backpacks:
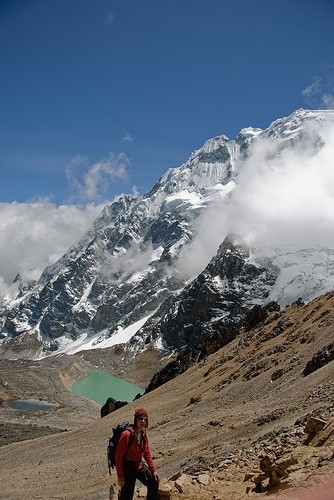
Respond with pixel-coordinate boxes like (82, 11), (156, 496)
(107, 420), (135, 475)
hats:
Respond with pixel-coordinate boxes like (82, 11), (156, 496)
(133, 408), (148, 429)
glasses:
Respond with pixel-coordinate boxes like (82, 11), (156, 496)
(138, 417), (147, 422)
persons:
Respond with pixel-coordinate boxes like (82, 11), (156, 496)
(115, 407), (160, 500)
